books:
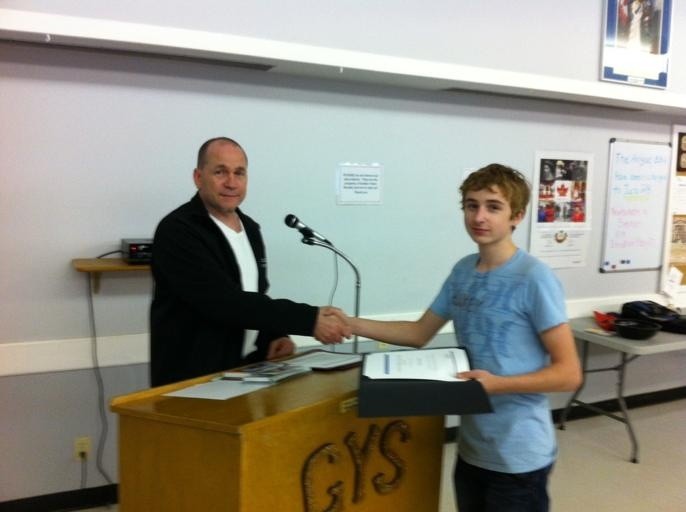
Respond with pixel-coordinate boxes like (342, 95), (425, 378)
(240, 362), (313, 383)
(220, 357), (286, 379)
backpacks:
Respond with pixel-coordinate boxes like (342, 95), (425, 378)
(625, 299), (686, 335)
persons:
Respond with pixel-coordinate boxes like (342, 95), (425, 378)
(309, 161), (586, 511)
(147, 136), (353, 398)
(538, 160), (585, 223)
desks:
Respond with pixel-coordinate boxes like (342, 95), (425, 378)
(556, 315), (686, 463)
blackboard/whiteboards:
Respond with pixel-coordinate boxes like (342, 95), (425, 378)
(599, 138), (672, 274)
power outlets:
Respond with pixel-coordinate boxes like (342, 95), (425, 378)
(76, 437), (90, 461)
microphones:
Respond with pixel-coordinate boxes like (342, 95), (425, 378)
(285, 214), (331, 245)
